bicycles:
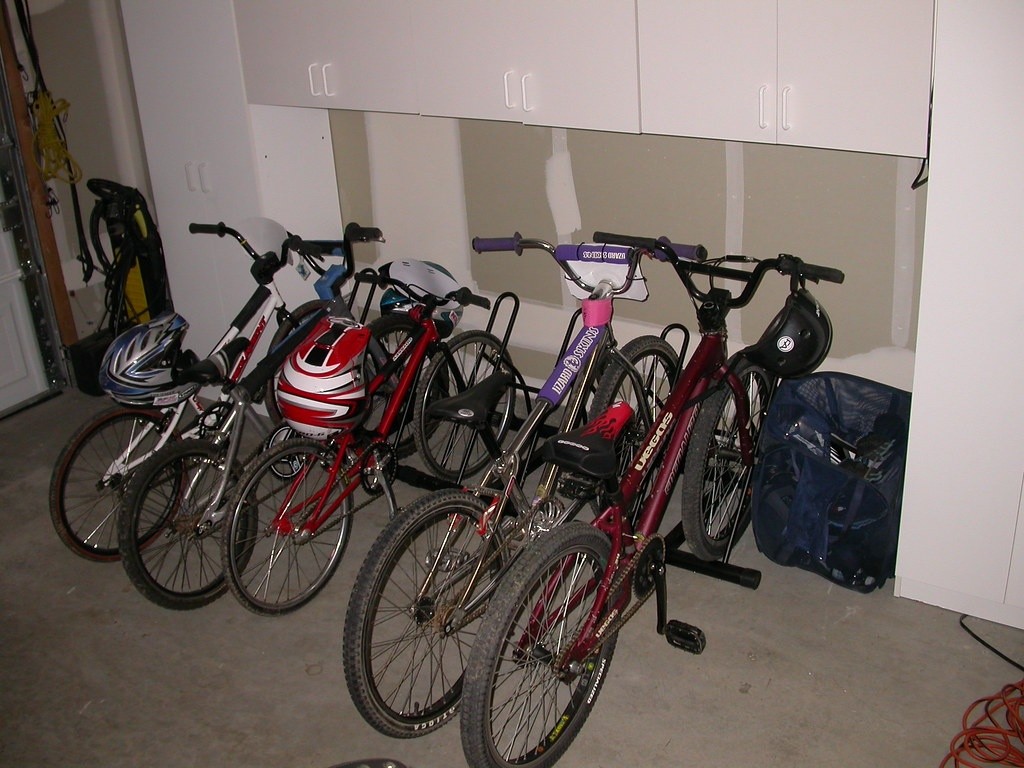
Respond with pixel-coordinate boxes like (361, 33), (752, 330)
(458, 230), (850, 767)
(340, 231), (708, 741)
(44, 220), (346, 562)
(220, 264), (522, 622)
(121, 221), (449, 611)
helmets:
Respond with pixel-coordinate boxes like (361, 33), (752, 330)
(272, 315), (370, 444)
(740, 288), (836, 380)
(98, 312), (205, 411)
(379, 261), (463, 337)
